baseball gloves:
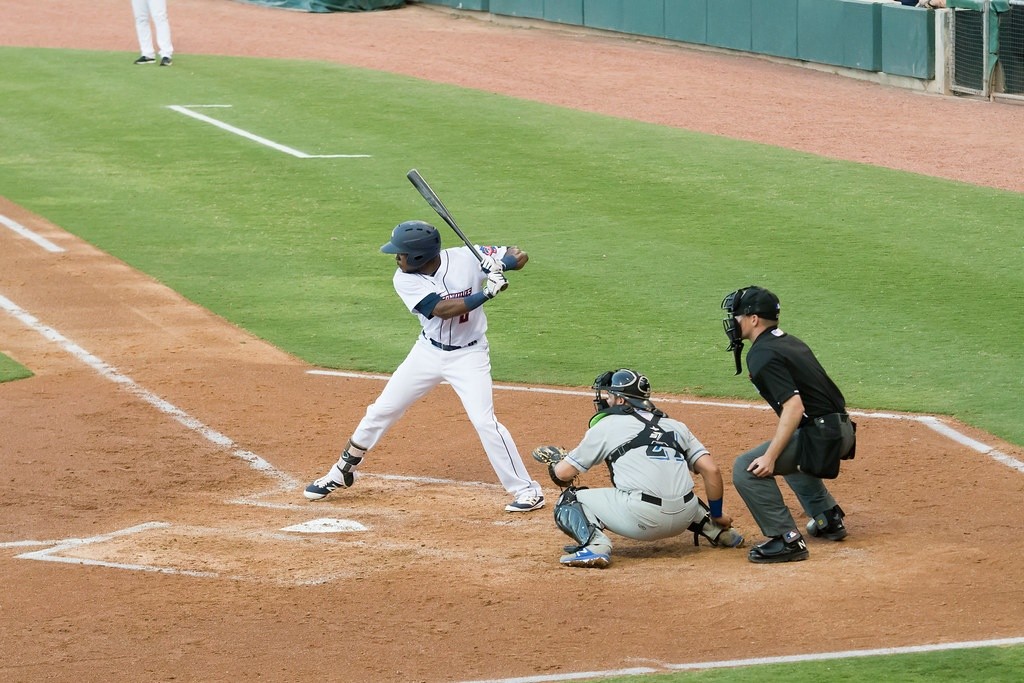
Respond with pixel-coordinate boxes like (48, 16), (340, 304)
(532, 445), (567, 465)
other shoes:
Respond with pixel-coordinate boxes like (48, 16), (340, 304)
(748, 534), (809, 563)
(806, 510), (847, 540)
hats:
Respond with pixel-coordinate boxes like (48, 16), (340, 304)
(730, 286), (780, 312)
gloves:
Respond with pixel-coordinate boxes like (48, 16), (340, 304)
(483, 271), (510, 299)
(479, 256), (506, 275)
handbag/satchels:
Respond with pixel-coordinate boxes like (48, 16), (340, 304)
(800, 416), (843, 479)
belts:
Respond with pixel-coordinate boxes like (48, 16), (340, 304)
(422, 330), (477, 352)
(839, 414), (849, 423)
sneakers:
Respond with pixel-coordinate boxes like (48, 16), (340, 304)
(135, 56), (156, 64)
(160, 57), (172, 66)
(716, 526), (744, 547)
(559, 545), (612, 568)
(504, 495), (545, 512)
(304, 473), (345, 500)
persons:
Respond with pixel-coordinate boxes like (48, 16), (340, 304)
(721, 285), (858, 564)
(303, 221), (546, 512)
(533, 367), (745, 569)
(131, 0), (174, 68)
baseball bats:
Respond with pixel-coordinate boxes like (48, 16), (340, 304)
(406, 169), (509, 291)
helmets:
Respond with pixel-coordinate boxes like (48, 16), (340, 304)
(594, 366), (651, 399)
(379, 220), (441, 269)
